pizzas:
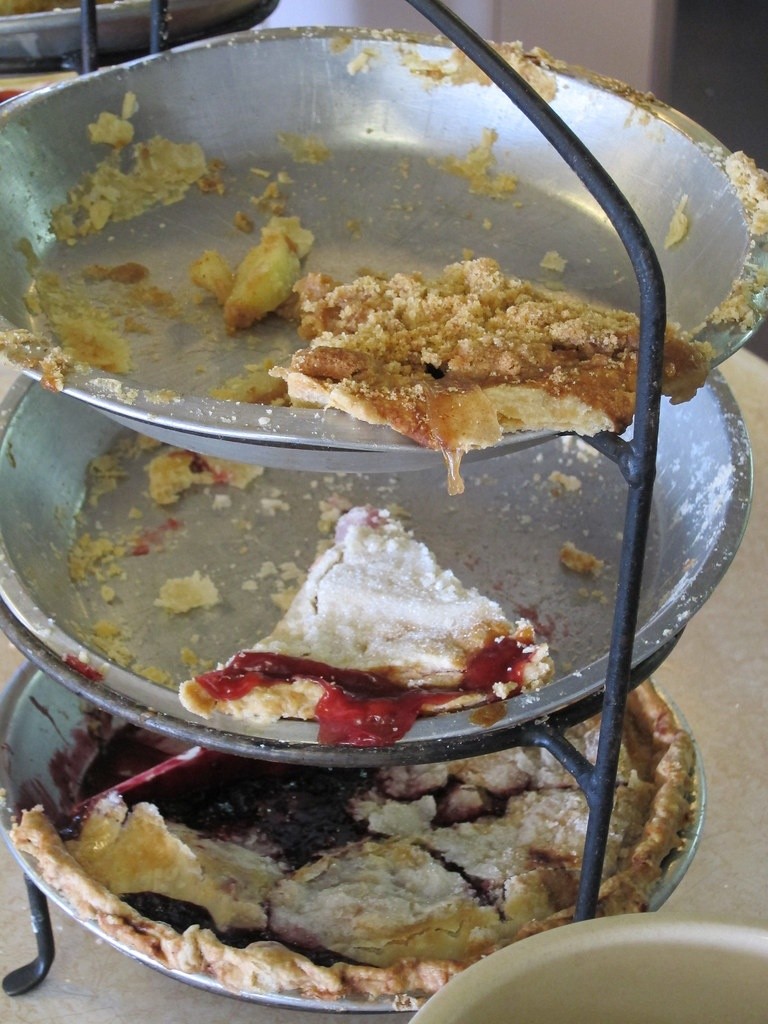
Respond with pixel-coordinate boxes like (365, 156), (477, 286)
(177, 504), (556, 746)
(267, 253), (706, 451)
(11, 673), (693, 1001)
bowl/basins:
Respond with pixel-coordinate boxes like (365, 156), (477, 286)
(0, 369), (756, 746)
(0, 21), (768, 477)
(0, 661), (709, 1015)
(406, 911), (767, 1023)
